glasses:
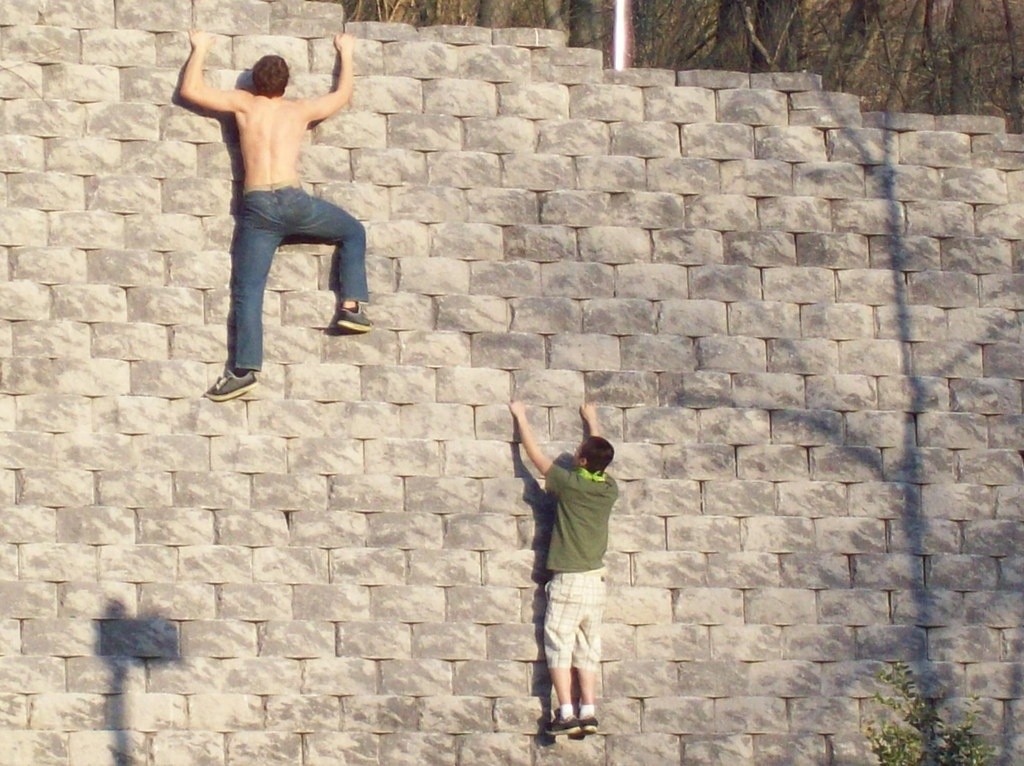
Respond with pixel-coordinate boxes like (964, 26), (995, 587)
(573, 443), (581, 455)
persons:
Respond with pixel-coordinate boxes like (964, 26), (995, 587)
(176, 30), (375, 402)
(506, 399), (620, 737)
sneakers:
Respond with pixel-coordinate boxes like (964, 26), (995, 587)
(544, 715), (582, 736)
(569, 716), (599, 737)
(337, 302), (374, 333)
(204, 370), (259, 401)
